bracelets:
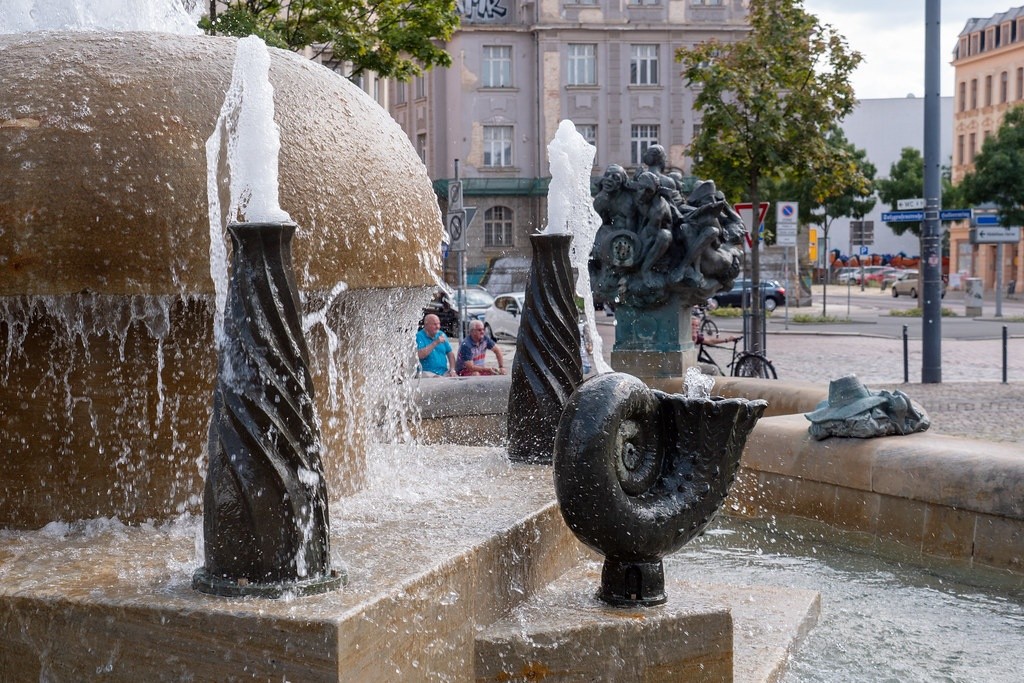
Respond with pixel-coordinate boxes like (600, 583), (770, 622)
(725, 338), (728, 343)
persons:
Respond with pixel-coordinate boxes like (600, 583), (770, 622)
(690, 317), (737, 376)
(417, 313), (455, 378)
(454, 320), (507, 377)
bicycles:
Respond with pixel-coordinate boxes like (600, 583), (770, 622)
(691, 304), (718, 341)
(697, 334), (779, 381)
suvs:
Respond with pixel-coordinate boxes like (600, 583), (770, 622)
(425, 282), (495, 339)
(699, 278), (787, 311)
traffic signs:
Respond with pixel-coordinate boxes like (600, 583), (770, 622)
(975, 225), (1020, 243)
(897, 198), (925, 211)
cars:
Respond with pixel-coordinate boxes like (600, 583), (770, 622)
(484, 291), (587, 343)
(829, 267), (903, 287)
(891, 272), (946, 298)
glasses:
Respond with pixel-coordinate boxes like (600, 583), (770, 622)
(474, 328), (485, 331)
